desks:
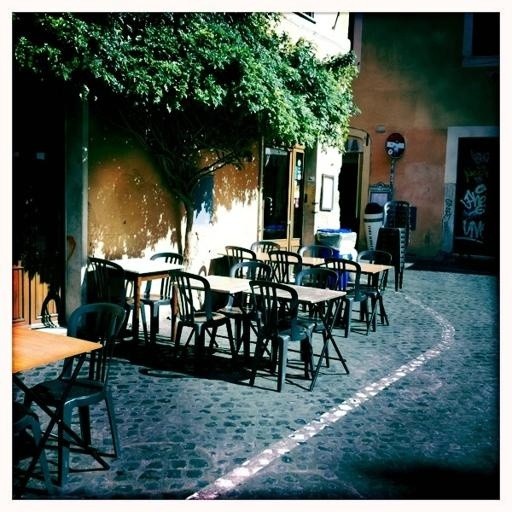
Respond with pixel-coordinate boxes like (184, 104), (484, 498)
(12, 323), (110, 500)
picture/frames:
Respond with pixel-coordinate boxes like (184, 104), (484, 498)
(320, 174), (335, 212)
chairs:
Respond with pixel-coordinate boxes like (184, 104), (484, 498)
(372, 200), (411, 292)
(12, 400), (57, 500)
(23, 302), (128, 490)
